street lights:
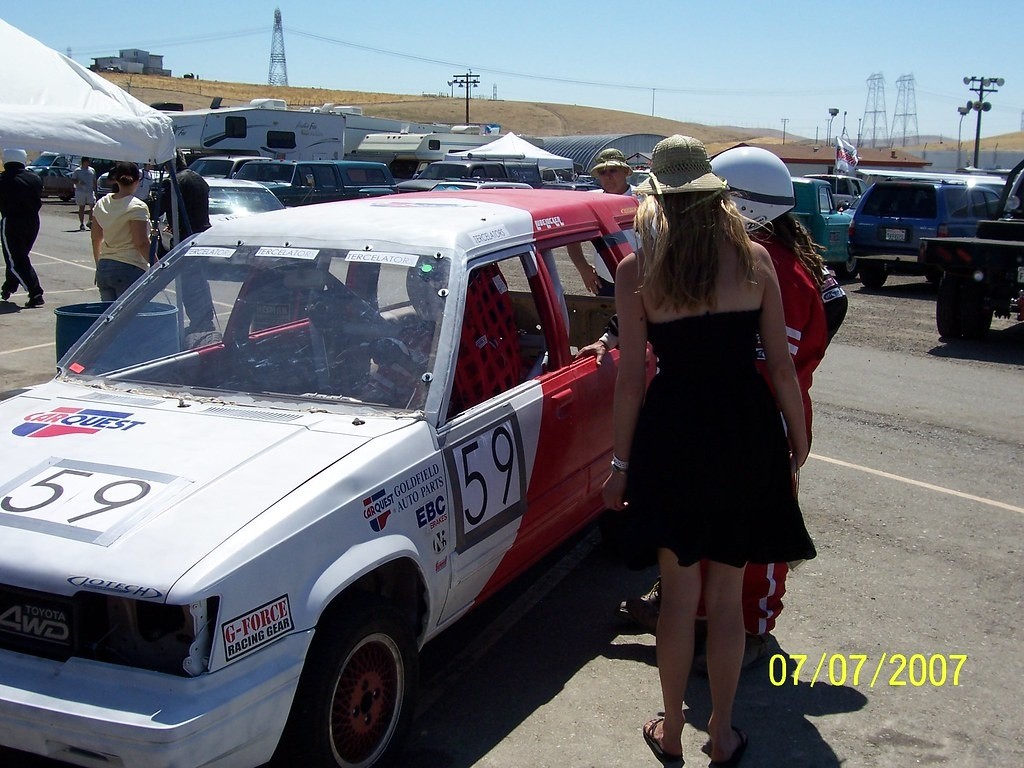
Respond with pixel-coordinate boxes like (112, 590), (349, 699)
(446, 75), (482, 124)
(827, 108), (840, 147)
(957, 107), (972, 171)
(963, 75), (1006, 166)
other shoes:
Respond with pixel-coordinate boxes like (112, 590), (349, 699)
(25, 294), (45, 307)
(86, 221), (92, 229)
(695, 634), (770, 672)
(1, 289), (11, 300)
(614, 575), (660, 629)
(80, 224), (85, 230)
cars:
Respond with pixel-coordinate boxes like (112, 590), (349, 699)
(0, 188), (656, 768)
(803, 173), (872, 220)
(788, 176), (858, 281)
(30, 107), (651, 275)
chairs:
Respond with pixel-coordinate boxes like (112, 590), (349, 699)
(405, 256), (525, 417)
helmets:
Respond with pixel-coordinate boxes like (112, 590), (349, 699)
(708, 146), (796, 231)
(2, 149), (28, 167)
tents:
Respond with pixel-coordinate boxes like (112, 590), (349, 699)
(0, 20), (175, 163)
(444, 132), (573, 172)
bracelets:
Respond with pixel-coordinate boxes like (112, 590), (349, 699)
(612, 453), (629, 473)
(75, 181), (80, 185)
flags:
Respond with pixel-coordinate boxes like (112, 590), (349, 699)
(836, 137), (859, 177)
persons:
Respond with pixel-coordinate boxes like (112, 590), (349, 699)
(575, 220), (848, 368)
(598, 133), (817, 768)
(696, 146), (824, 680)
(567, 147), (642, 297)
(158, 146), (216, 334)
(133, 164), (153, 200)
(0, 149), (45, 308)
(105, 161), (122, 193)
(91, 162), (159, 301)
(71, 156), (97, 232)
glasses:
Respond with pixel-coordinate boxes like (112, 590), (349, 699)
(598, 168), (623, 175)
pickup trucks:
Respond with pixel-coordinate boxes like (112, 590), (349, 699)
(918, 159), (1024, 340)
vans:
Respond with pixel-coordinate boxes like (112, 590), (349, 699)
(847, 177), (1003, 288)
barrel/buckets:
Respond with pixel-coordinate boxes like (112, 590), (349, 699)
(54, 300), (181, 375)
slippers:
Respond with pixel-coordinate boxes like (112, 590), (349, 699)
(709, 726), (749, 768)
(643, 717), (685, 762)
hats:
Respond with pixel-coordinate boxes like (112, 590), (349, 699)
(632, 134), (730, 194)
(591, 148), (633, 179)
